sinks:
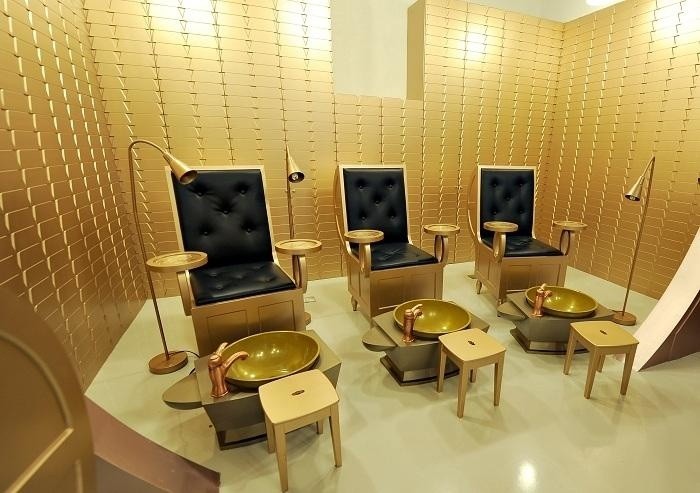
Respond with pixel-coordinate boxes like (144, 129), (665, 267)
(525, 286), (598, 318)
(224, 330), (320, 392)
(394, 298), (471, 339)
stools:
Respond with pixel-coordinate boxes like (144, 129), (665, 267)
(258, 368), (343, 492)
(436, 326), (506, 417)
(564, 319), (639, 398)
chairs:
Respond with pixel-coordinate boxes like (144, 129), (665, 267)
(465, 165), (588, 317)
(333, 163), (460, 327)
(163, 164), (307, 355)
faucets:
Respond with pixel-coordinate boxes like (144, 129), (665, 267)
(206, 343), (249, 397)
(534, 283), (552, 316)
(402, 303), (422, 342)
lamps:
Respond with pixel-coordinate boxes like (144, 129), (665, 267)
(128, 139), (198, 376)
(285, 145), (311, 323)
(612, 155), (656, 325)
